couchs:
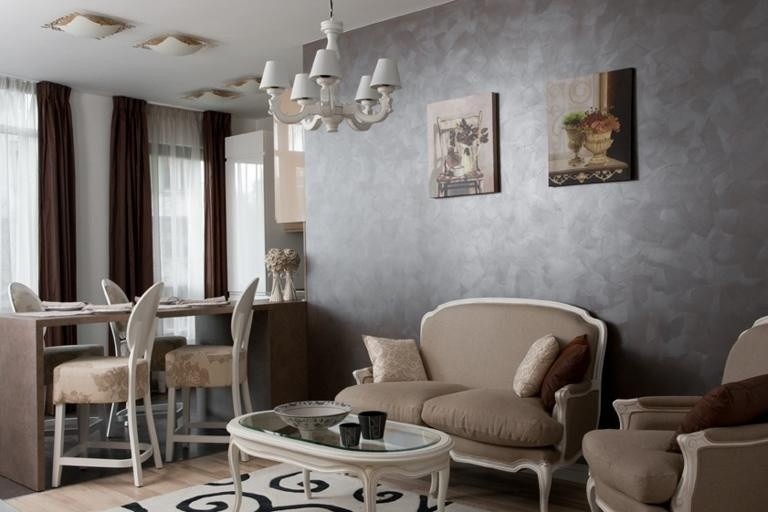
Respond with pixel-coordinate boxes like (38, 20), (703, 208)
(582, 315), (768, 511)
(335, 297), (608, 511)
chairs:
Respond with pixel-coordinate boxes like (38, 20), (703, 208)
(101, 278), (187, 437)
(53, 282), (163, 487)
(9, 281), (105, 442)
(166, 278), (259, 462)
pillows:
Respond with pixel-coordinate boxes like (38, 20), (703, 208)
(361, 335), (428, 383)
(542, 334), (591, 409)
(665, 375), (768, 453)
(513, 332), (560, 398)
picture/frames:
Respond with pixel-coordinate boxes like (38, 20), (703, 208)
(425, 92), (497, 199)
(548, 67), (636, 187)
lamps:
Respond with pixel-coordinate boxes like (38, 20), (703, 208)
(221, 78), (267, 94)
(132, 32), (218, 57)
(259, 1), (402, 133)
(39, 11), (136, 40)
(179, 90), (245, 105)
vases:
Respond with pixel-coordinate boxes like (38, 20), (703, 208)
(284, 271), (297, 301)
(270, 270), (283, 302)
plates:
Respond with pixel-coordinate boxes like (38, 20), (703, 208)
(158, 298), (178, 305)
(187, 302), (231, 309)
(44, 306), (84, 312)
(90, 310), (131, 315)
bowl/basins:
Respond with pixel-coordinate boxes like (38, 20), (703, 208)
(273, 399), (352, 433)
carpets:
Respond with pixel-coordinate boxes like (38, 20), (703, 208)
(107, 463), (495, 512)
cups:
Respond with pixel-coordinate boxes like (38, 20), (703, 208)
(338, 422), (362, 449)
(357, 410), (387, 441)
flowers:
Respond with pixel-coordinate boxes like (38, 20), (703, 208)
(283, 248), (301, 272)
(264, 248), (287, 273)
